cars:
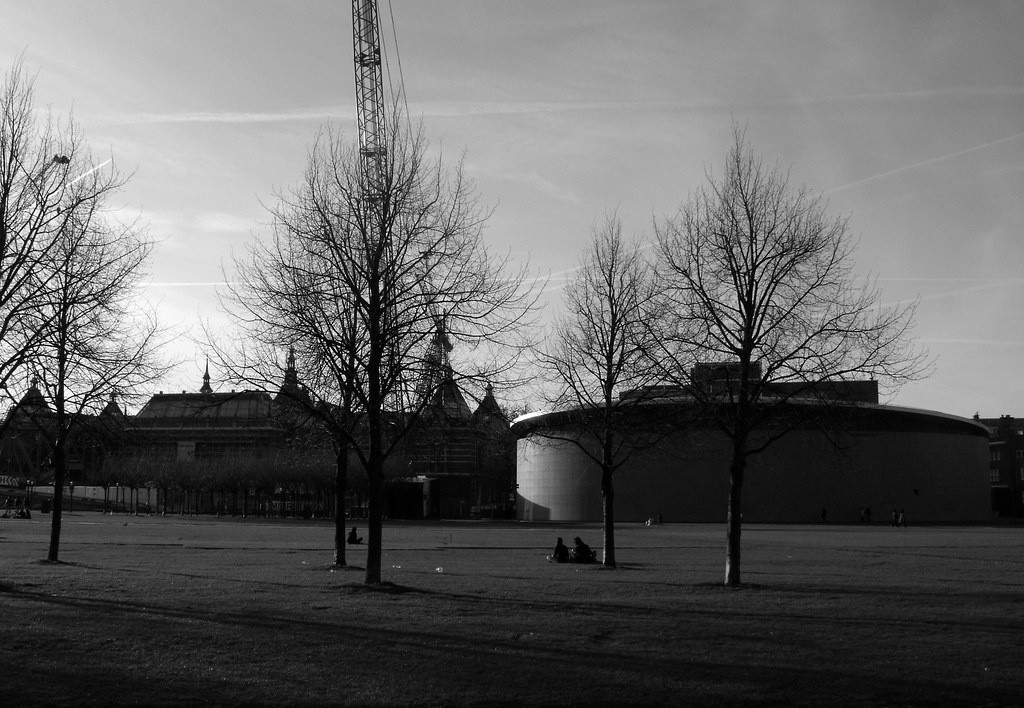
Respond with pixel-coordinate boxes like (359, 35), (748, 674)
(345, 506), (368, 517)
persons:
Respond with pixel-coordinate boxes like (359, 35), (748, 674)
(740, 512), (744, 523)
(859, 505), (871, 525)
(551, 537), (592, 562)
(658, 512), (663, 525)
(347, 527), (363, 544)
(888, 508), (907, 527)
(5, 496), (31, 519)
(821, 505), (828, 524)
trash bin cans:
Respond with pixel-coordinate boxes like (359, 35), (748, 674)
(41, 499), (50, 514)
